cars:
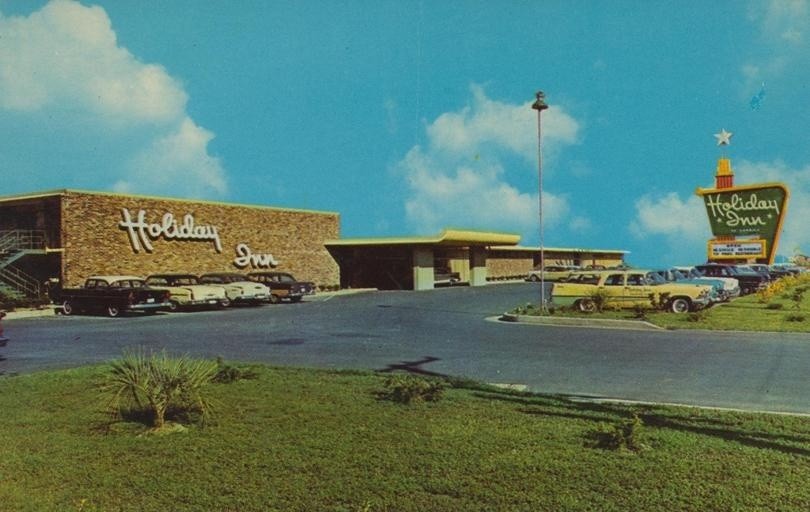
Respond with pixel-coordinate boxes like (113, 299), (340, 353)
(246, 272), (316, 304)
(200, 272), (271, 308)
(144, 274), (229, 312)
(549, 269), (711, 315)
(48, 276), (173, 318)
(528, 262), (810, 308)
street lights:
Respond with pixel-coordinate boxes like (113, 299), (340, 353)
(532, 92), (548, 314)
